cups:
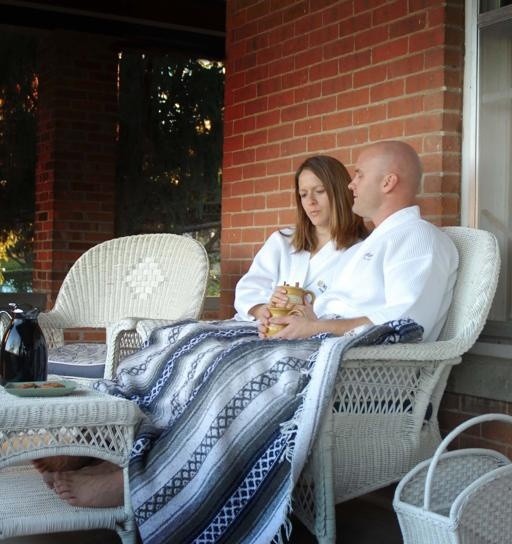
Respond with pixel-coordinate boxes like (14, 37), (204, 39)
(266, 306), (305, 338)
(275, 286), (316, 310)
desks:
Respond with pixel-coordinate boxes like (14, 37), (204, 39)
(0, 385), (141, 544)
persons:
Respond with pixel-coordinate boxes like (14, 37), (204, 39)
(41, 139), (462, 511)
(28, 152), (374, 468)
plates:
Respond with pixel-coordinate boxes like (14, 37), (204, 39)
(3, 378), (78, 398)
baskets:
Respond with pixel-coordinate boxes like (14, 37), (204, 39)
(392, 414), (511, 544)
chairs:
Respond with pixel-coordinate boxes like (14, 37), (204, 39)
(136, 225), (502, 544)
(0, 232), (211, 380)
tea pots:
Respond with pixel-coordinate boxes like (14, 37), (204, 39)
(6, 304), (44, 382)
